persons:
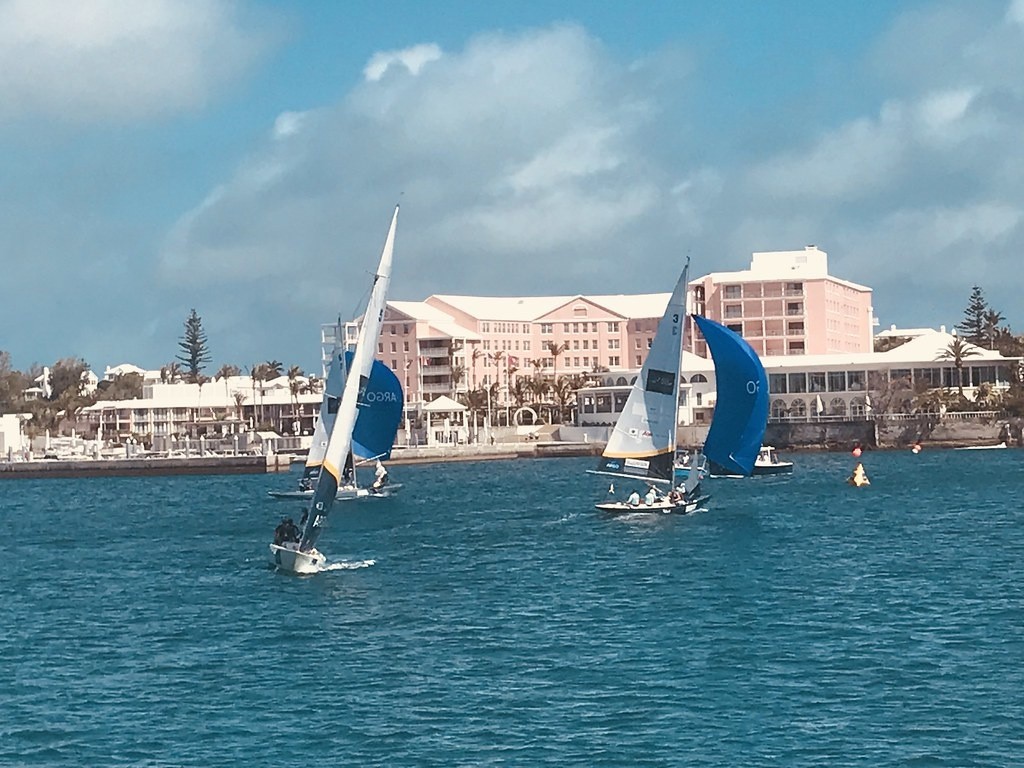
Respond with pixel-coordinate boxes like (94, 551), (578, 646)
(645, 486), (657, 506)
(275, 518), (300, 546)
(667, 482), (686, 503)
(626, 490), (640, 506)
(299, 477), (313, 492)
(759, 451), (779, 464)
(676, 453), (690, 468)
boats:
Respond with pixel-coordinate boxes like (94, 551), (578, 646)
(673, 448), (709, 478)
(708, 442), (795, 479)
(846, 462), (872, 489)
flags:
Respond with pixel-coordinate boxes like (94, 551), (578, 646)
(423, 357), (432, 367)
(508, 354), (518, 366)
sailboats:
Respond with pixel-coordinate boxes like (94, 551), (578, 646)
(583, 257), (770, 516)
(267, 202), (402, 576)
(266, 312), (402, 502)
